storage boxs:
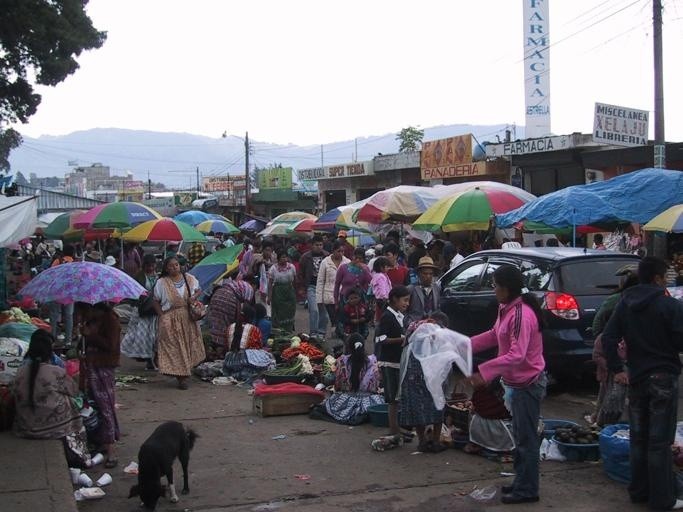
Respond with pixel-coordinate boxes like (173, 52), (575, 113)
(251, 392), (325, 420)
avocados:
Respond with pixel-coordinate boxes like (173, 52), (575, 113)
(551, 422), (600, 443)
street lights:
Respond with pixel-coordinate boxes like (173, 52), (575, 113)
(221, 130), (249, 222)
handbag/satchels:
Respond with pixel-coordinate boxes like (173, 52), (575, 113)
(138, 296), (156, 315)
(189, 300), (207, 320)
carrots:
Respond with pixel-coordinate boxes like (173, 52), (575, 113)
(282, 341), (322, 358)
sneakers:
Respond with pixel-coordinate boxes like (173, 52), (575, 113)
(502, 496), (539, 503)
(501, 485), (515, 493)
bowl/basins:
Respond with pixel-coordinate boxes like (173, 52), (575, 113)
(264, 374), (296, 384)
(367, 405), (401, 426)
(542, 419), (578, 434)
(550, 434), (600, 461)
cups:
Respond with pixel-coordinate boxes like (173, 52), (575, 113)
(95, 473), (112, 486)
(89, 452), (103, 465)
(78, 473), (92, 487)
(68, 468), (79, 484)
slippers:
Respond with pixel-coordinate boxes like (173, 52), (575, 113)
(106, 456), (118, 468)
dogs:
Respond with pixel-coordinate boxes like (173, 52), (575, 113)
(127, 420), (202, 512)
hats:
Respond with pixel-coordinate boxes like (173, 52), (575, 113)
(417, 257), (438, 269)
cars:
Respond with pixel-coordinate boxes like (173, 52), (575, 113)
(137, 236), (223, 271)
(429, 241), (643, 395)
